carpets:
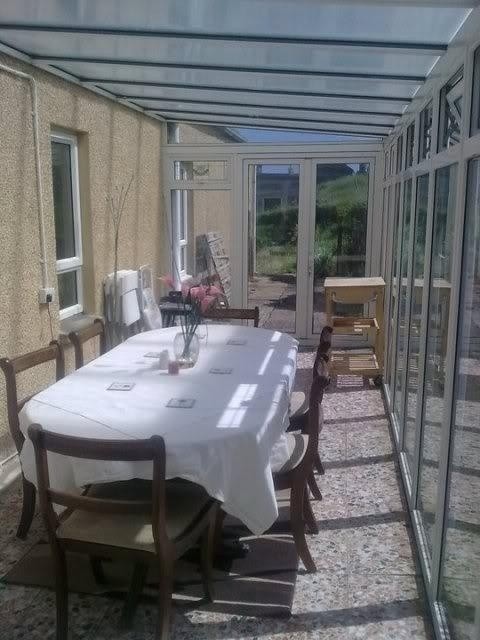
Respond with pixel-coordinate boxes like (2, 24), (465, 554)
(1, 522), (302, 620)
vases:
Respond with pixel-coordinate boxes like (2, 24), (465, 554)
(173, 333), (200, 369)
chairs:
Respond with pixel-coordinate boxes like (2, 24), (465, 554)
(0, 339), (87, 540)
(208, 306), (261, 329)
(289, 325), (334, 500)
(218, 353), (329, 576)
(102, 264), (162, 349)
(68, 316), (110, 370)
(27, 421), (221, 640)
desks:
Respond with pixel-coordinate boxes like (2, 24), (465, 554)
(159, 301), (194, 327)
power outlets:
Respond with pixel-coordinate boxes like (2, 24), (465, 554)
(39, 288), (55, 304)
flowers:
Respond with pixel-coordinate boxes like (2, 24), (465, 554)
(160, 273), (227, 358)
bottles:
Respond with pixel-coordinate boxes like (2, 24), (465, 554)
(159, 349), (181, 376)
